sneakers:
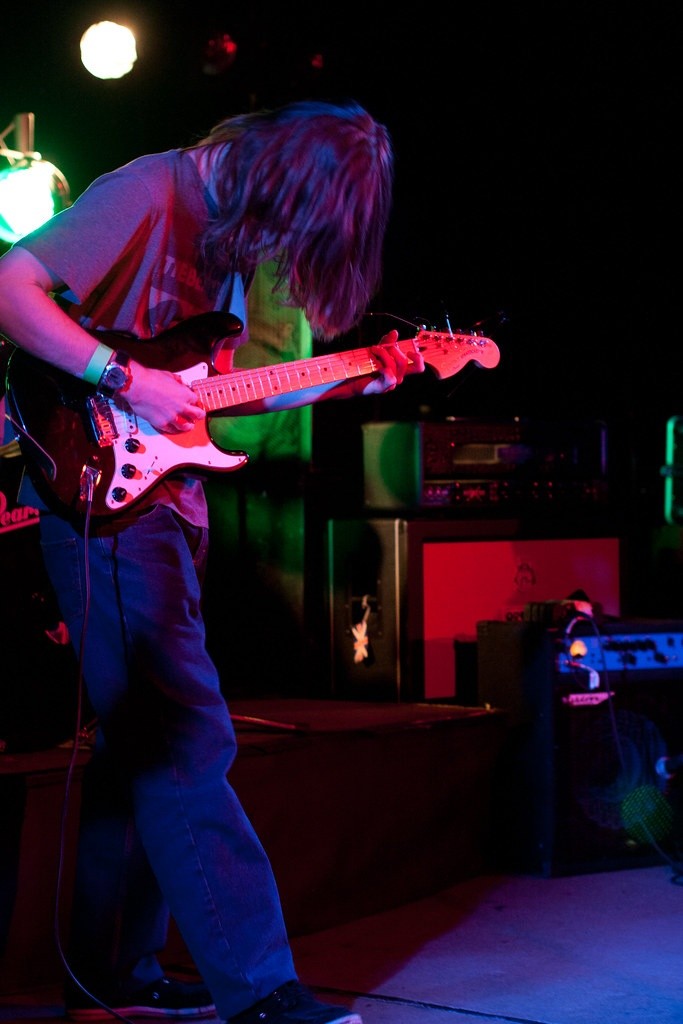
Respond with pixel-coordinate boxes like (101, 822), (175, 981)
(218, 979), (362, 1024)
(62, 970), (215, 1021)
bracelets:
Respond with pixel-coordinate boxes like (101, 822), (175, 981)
(82, 342), (114, 386)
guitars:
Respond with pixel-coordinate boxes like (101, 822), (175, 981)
(5, 309), (502, 524)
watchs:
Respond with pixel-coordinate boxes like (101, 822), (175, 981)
(99, 349), (131, 399)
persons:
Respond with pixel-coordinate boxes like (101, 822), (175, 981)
(0, 95), (425, 1024)
